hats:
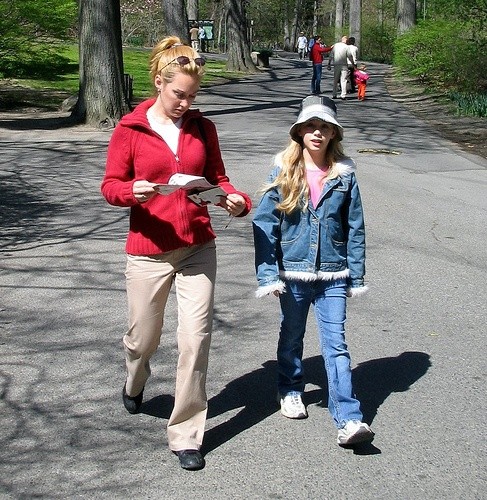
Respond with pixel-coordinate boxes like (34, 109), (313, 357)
(289, 95), (344, 148)
(300, 31), (305, 34)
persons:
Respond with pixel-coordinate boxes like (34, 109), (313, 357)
(353, 64), (369, 100)
(101, 35), (252, 470)
(197, 25), (206, 53)
(295, 31), (308, 61)
(327, 35), (356, 100)
(189, 23), (199, 53)
(252, 95), (376, 446)
(309, 35), (334, 95)
(309, 35), (314, 52)
(347, 37), (358, 94)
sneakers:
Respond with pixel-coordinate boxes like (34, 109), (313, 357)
(172, 449), (203, 469)
(337, 419), (375, 447)
(276, 390), (308, 419)
(122, 380), (144, 414)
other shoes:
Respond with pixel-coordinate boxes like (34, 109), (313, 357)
(332, 95), (337, 99)
(340, 97), (345, 100)
(312, 90), (323, 94)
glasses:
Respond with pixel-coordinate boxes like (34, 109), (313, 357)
(157, 56), (207, 73)
